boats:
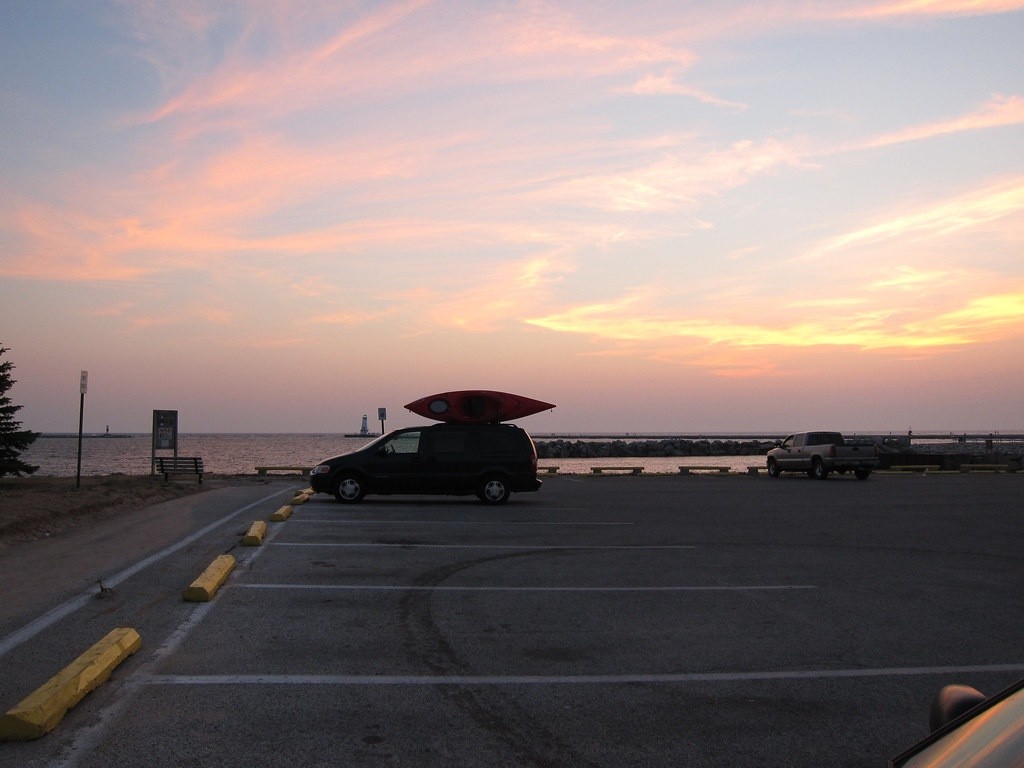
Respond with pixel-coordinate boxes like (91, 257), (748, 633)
(343, 415), (382, 438)
(403, 389), (557, 424)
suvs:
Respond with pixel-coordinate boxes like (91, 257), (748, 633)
(309, 422), (543, 505)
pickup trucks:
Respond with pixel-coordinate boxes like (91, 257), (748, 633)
(766, 431), (880, 480)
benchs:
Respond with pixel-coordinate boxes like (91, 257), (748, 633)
(155, 457), (213, 483)
(537, 464), (768, 476)
(254, 466), (314, 477)
(891, 462), (1024, 473)
